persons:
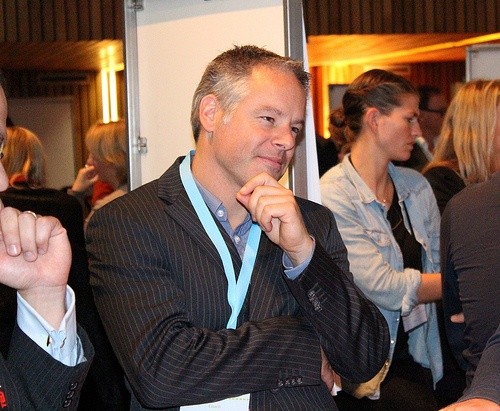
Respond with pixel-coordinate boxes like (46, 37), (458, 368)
(85, 45), (390, 411)
(319, 69), (444, 411)
(441, 174), (500, 396)
(66, 119), (130, 231)
(423, 80), (500, 214)
(0, 84), (95, 411)
(1, 126), (66, 198)
(326, 108), (350, 165)
(392, 84), (445, 173)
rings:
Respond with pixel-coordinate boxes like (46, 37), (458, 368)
(23, 209), (39, 219)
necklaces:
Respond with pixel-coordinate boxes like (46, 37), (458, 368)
(378, 195), (390, 204)
